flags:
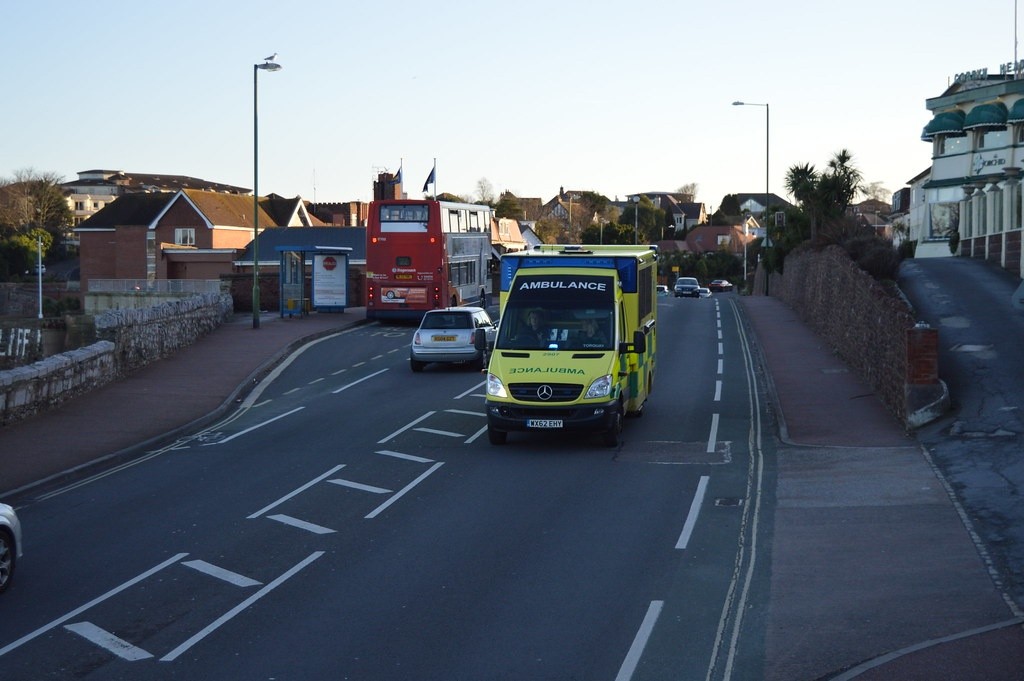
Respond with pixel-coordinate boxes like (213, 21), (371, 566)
(389, 166), (402, 184)
(422, 166), (436, 192)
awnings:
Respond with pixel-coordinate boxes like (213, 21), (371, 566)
(1006, 97), (1024, 123)
(963, 101), (1008, 129)
(920, 108), (966, 140)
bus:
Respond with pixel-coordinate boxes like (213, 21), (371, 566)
(364, 198), (493, 323)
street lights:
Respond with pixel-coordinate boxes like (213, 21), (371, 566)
(252, 63), (284, 328)
(567, 192), (581, 224)
(732, 100), (770, 295)
(599, 217), (610, 245)
(634, 196), (640, 245)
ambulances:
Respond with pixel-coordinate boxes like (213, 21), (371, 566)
(484, 243), (658, 448)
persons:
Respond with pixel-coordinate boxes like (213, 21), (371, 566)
(577, 315), (608, 344)
(510, 309), (549, 348)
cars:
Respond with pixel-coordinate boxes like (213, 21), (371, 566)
(409, 307), (493, 372)
(708, 279), (733, 292)
(699, 288), (712, 298)
(35, 264), (46, 276)
(657, 285), (670, 296)
(674, 276), (700, 298)
(0, 503), (23, 589)
